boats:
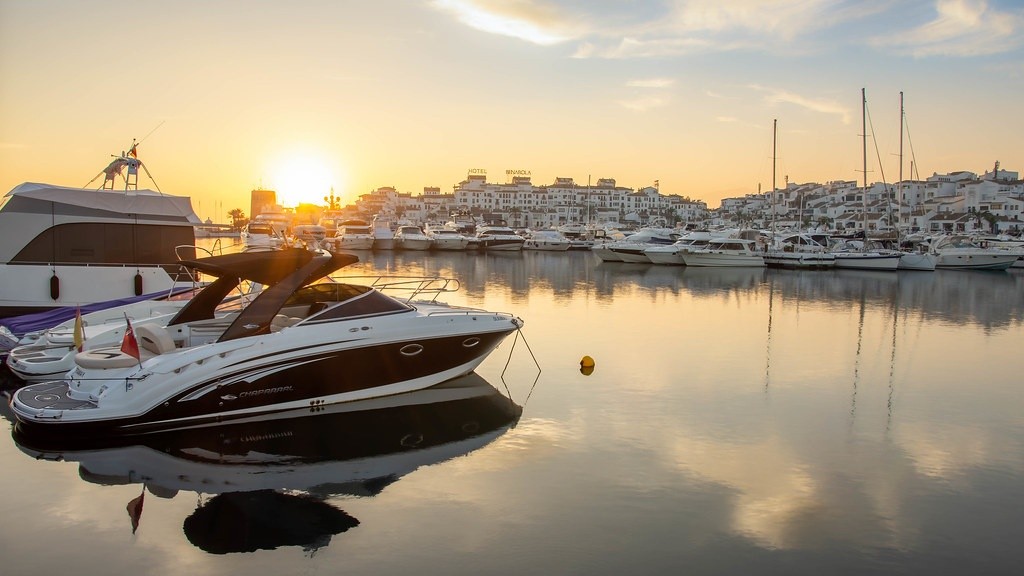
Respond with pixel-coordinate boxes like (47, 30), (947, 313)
(609, 229), (683, 262)
(644, 231), (732, 265)
(515, 225), (623, 248)
(592, 240), (624, 261)
(467, 218), (524, 250)
(9, 247), (524, 438)
(979, 236), (1024, 247)
(237, 207), (341, 249)
(12, 371), (538, 554)
(524, 230), (570, 250)
(0, 139), (199, 317)
(680, 222), (935, 235)
(929, 234), (1024, 269)
(445, 210), (477, 249)
(394, 226), (433, 250)
(0, 280), (269, 383)
(423, 223), (468, 250)
(681, 238), (768, 267)
(334, 220), (374, 250)
(370, 215), (396, 250)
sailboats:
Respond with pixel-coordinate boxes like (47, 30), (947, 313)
(763, 118), (835, 268)
(865, 91), (939, 272)
(830, 88), (903, 270)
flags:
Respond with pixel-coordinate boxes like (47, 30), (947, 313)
(130, 144), (137, 158)
(73, 309), (82, 352)
(120, 322), (140, 360)
